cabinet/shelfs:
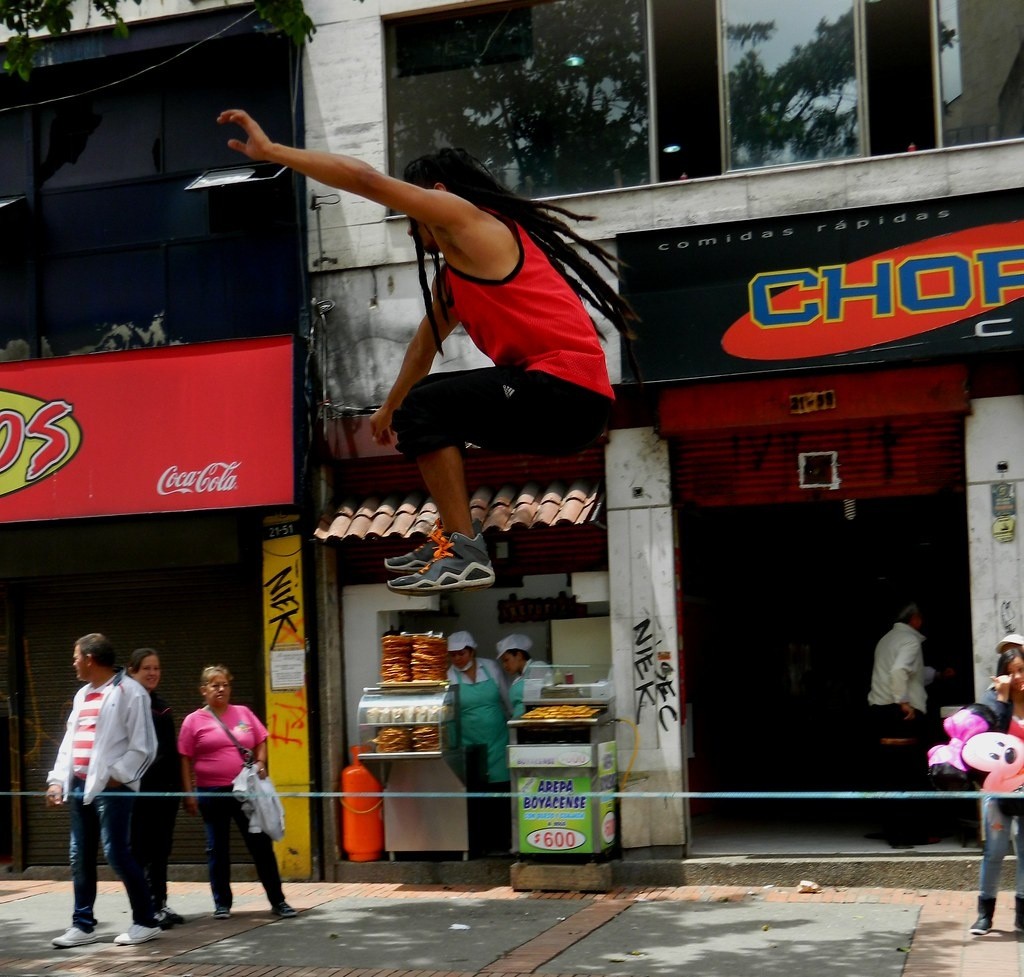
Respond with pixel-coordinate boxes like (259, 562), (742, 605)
(355, 685), (459, 760)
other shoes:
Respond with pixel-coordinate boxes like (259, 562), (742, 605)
(213, 906), (230, 918)
(271, 901), (297, 916)
(145, 905), (185, 930)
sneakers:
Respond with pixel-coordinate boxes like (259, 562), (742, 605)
(384, 518), (483, 574)
(113, 924), (162, 944)
(51, 926), (99, 947)
(387, 532), (497, 596)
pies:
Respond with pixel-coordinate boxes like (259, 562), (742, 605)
(521, 704), (600, 718)
(371, 725), (449, 753)
(379, 634), (449, 682)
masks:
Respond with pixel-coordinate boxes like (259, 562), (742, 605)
(454, 660), (473, 671)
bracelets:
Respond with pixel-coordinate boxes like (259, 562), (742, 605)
(254, 760), (266, 767)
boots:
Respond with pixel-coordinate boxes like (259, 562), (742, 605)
(1013, 896), (1024, 933)
(969, 895), (996, 934)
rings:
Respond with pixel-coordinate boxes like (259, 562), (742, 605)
(260, 768), (265, 772)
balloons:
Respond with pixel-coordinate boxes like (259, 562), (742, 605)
(925, 703), (1024, 819)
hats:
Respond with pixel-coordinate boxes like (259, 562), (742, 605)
(496, 633), (534, 659)
(995, 634), (1024, 654)
(447, 631), (477, 651)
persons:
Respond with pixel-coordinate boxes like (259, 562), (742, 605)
(125, 648), (185, 931)
(45, 633), (163, 947)
(446, 630), (514, 855)
(177, 665), (298, 918)
(867, 603), (956, 845)
(494, 634), (555, 720)
(215, 109), (643, 597)
(968, 634), (1024, 935)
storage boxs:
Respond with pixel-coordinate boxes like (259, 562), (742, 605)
(541, 686), (592, 699)
(523, 678), (545, 699)
(592, 681), (611, 698)
